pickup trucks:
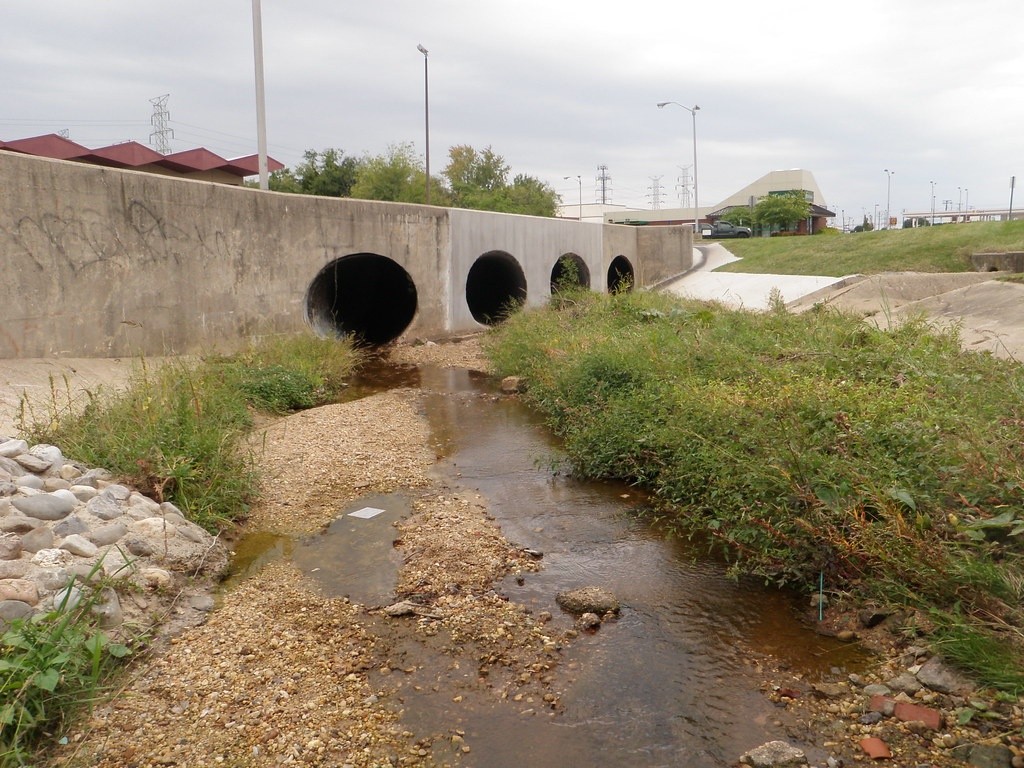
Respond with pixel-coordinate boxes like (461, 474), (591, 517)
(699, 220), (752, 238)
(693, 222), (713, 233)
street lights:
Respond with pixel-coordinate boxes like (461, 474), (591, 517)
(415, 44), (432, 205)
(884, 168), (895, 212)
(957, 186), (961, 224)
(929, 180), (937, 226)
(656, 100), (701, 233)
(964, 188), (968, 221)
(563, 175), (582, 221)
